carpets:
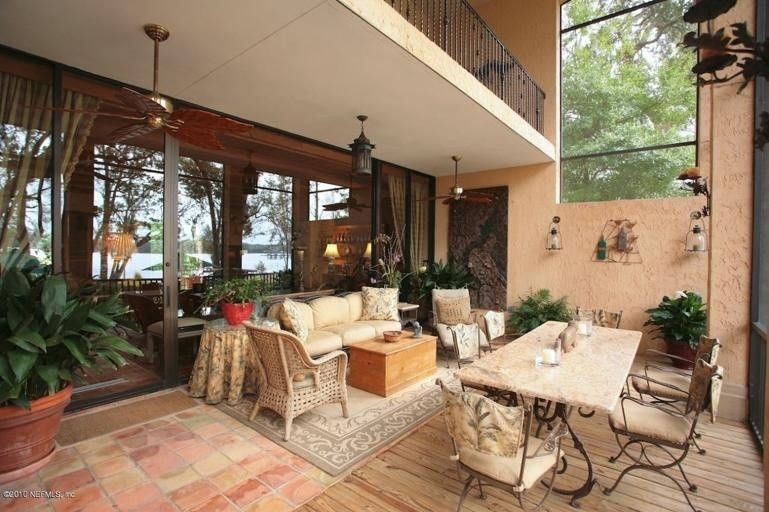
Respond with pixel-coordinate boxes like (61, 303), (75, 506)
(213, 364), (476, 477)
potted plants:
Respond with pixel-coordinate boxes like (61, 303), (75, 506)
(202, 278), (265, 325)
(0, 248), (145, 484)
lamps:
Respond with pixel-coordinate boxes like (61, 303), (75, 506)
(684, 216), (711, 252)
(241, 151), (258, 194)
(323, 243), (340, 273)
(545, 216), (562, 250)
(346, 115), (376, 177)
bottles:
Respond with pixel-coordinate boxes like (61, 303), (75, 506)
(618, 226), (628, 251)
(598, 236), (606, 259)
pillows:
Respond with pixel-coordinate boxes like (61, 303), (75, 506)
(266, 301), (313, 331)
(360, 287), (400, 322)
(280, 297), (308, 344)
(343, 291), (362, 322)
(309, 295), (349, 330)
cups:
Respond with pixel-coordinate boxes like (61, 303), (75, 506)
(534, 334), (562, 367)
(571, 309), (593, 337)
(415, 326), (422, 336)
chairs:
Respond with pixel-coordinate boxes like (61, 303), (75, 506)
(246, 326), (349, 442)
(123, 293), (164, 358)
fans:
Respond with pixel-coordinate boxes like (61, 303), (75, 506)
(416, 155), (495, 204)
(322, 176), (372, 212)
(22, 22), (256, 150)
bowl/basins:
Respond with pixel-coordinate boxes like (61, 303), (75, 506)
(383, 330), (401, 342)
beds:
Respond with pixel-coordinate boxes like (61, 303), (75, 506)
(146, 317), (207, 364)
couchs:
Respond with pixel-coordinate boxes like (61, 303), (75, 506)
(263, 291), (402, 369)
